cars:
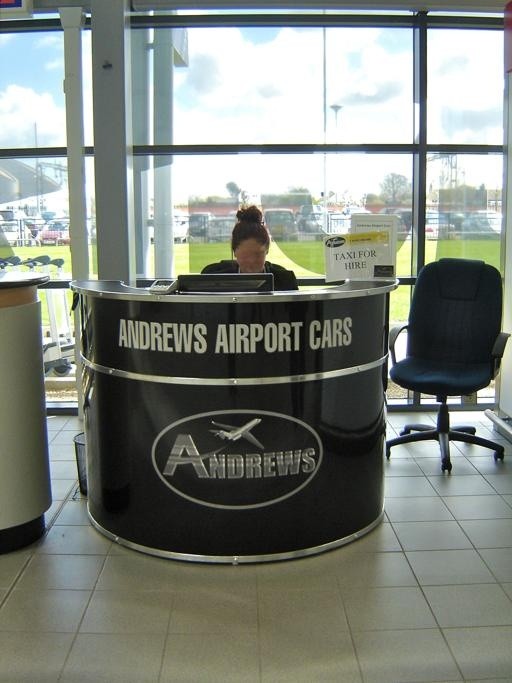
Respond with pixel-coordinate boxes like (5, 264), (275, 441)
(0, 210), (91, 245)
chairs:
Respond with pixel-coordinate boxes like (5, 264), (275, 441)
(386, 258), (511, 477)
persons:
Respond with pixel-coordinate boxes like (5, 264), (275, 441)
(200, 203), (298, 292)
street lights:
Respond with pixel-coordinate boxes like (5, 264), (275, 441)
(330, 101), (344, 202)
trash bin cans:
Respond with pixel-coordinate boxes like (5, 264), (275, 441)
(74, 432), (87, 495)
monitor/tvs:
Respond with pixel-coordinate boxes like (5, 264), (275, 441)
(177, 273), (275, 293)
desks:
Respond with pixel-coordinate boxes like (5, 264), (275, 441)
(1, 270), (52, 555)
